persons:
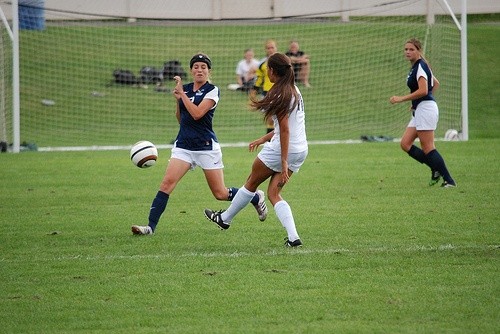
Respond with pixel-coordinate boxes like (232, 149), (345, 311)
(204, 53), (309, 248)
(228, 37), (311, 133)
(389, 39), (457, 188)
(132, 52), (268, 237)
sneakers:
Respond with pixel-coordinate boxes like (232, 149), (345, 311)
(441, 181), (457, 188)
(204, 208), (230, 230)
(430, 169), (441, 186)
(285, 237), (302, 247)
(131, 224), (153, 235)
(254, 190), (268, 222)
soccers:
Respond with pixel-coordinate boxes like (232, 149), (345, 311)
(130, 140), (158, 168)
